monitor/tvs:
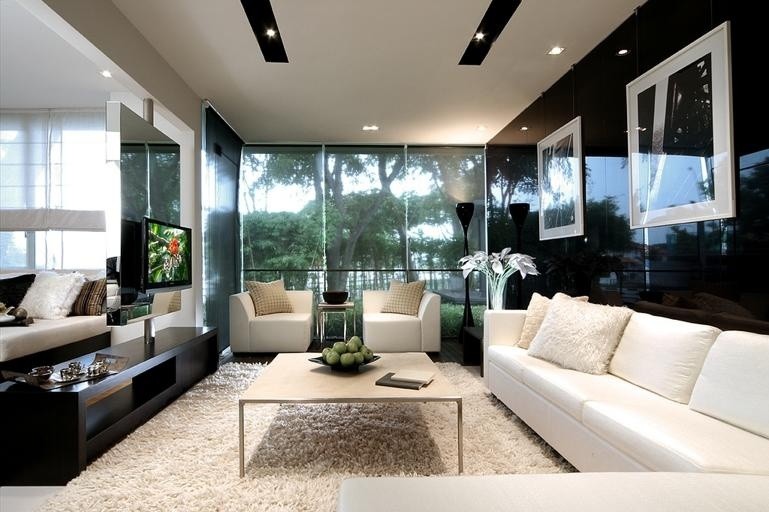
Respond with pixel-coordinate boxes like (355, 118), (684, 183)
(142, 216), (193, 295)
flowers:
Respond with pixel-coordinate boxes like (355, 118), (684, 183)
(459, 244), (541, 311)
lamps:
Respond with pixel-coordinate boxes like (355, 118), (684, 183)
(456, 202), (475, 342)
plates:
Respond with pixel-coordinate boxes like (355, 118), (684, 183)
(306, 355), (382, 372)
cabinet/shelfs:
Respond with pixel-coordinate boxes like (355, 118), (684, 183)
(14, 328), (221, 487)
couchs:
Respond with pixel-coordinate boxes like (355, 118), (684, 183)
(482, 309), (768, 473)
(0, 268), (111, 374)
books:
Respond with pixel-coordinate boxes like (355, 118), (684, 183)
(375, 369), (435, 391)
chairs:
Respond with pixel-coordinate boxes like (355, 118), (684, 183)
(228, 278), (315, 357)
(363, 278), (441, 354)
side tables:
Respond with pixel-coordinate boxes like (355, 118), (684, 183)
(317, 304), (357, 351)
(462, 325), (485, 375)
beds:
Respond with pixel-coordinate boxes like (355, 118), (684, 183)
(338, 472), (767, 512)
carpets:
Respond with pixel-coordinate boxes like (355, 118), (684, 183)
(42, 361), (580, 512)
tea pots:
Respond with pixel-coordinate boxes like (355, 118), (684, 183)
(86, 360), (111, 375)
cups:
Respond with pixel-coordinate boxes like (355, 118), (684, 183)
(69, 361), (82, 374)
(61, 368), (73, 382)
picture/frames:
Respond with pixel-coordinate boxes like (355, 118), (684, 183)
(625, 21), (736, 230)
(536, 115), (585, 241)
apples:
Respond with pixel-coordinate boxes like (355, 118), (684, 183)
(326, 351), (340, 364)
(332, 342), (347, 354)
(347, 340), (358, 352)
(322, 348), (330, 361)
(353, 352), (364, 363)
(358, 345), (366, 352)
(350, 336), (362, 348)
(340, 352), (354, 367)
(361, 349), (373, 360)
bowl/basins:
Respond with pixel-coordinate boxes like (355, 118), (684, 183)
(32, 365), (54, 373)
(323, 292), (348, 303)
(28, 370), (53, 383)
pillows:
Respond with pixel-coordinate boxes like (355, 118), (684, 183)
(17, 271), (83, 319)
(528, 293), (633, 376)
(517, 291), (587, 352)
(608, 311), (721, 404)
(243, 279), (295, 316)
(0, 275), (36, 312)
(380, 279), (427, 314)
(688, 330), (769, 437)
(65, 278), (106, 317)
